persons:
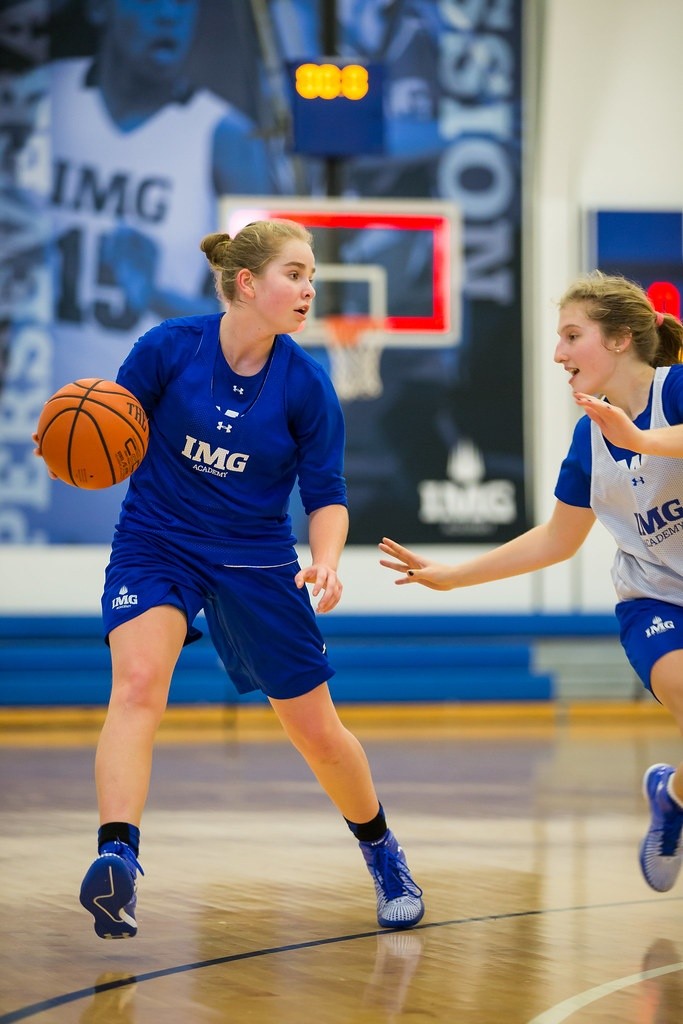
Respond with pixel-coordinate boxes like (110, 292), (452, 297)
(80, 218), (425, 941)
(377, 270), (683, 894)
(2, 0), (281, 538)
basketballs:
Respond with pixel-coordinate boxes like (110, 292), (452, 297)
(36, 377), (151, 491)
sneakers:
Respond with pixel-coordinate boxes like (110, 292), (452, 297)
(77, 842), (145, 939)
(360, 829), (425, 926)
(639, 763), (683, 893)
